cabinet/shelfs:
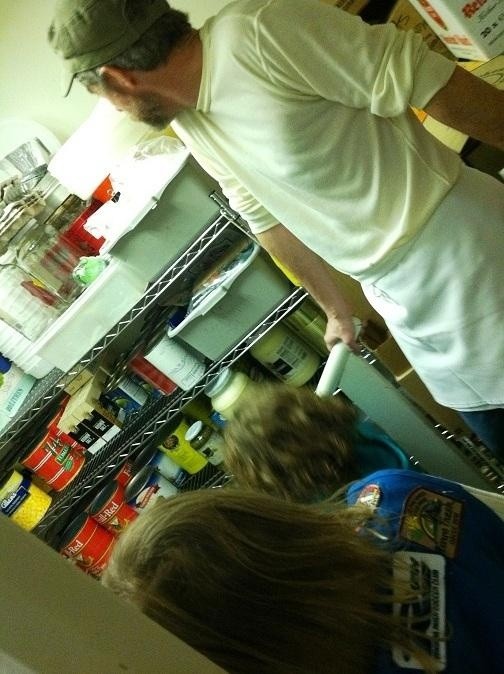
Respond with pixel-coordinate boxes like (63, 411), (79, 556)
(6, 186), (504, 582)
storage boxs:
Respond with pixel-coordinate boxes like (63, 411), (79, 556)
(409, 0), (503, 62)
(29, 257), (150, 375)
(100, 152), (226, 283)
(168, 239), (292, 361)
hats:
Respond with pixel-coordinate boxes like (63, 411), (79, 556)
(48, 0), (170, 99)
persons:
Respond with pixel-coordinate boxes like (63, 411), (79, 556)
(102, 488), (504, 673)
(206, 394), (504, 598)
(36, 0), (504, 451)
